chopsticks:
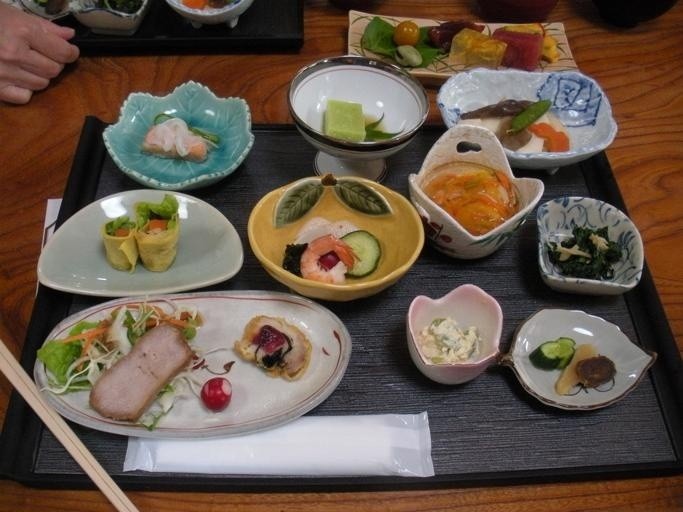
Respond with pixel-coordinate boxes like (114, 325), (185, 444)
(0, 338), (142, 512)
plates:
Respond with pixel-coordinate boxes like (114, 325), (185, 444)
(33, 289), (353, 438)
(408, 122), (544, 262)
(36, 187), (244, 296)
(69, 0), (146, 36)
(538, 197), (645, 294)
(31, 0), (78, 25)
(494, 305), (658, 418)
(164, 0), (254, 28)
(247, 174), (425, 302)
(102, 78), (254, 198)
(404, 283), (503, 387)
(438, 65), (618, 171)
(346, 10), (580, 93)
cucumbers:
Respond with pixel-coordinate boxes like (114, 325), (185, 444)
(532, 338), (575, 369)
(338, 231), (382, 277)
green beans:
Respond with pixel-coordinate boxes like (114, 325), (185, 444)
(506, 99), (551, 132)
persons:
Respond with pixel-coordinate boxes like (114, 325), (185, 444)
(0, 1), (80, 105)
